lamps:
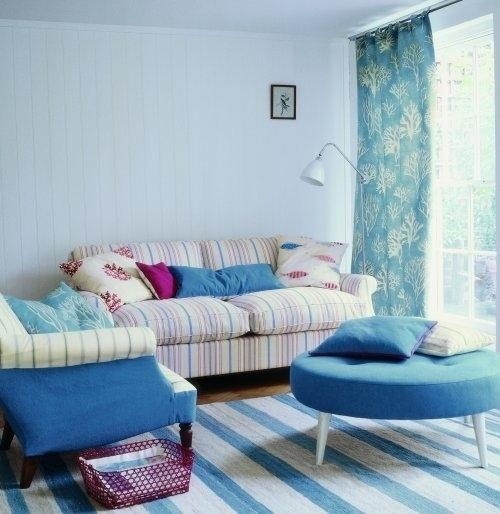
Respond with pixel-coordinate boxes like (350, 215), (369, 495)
(299, 140), (369, 274)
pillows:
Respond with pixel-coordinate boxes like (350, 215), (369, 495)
(134, 260), (179, 301)
(415, 318), (496, 358)
(164, 263), (288, 299)
(5, 282), (115, 335)
(306, 312), (441, 360)
(54, 251), (155, 315)
(273, 231), (351, 292)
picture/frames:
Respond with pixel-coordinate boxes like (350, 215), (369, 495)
(268, 84), (298, 121)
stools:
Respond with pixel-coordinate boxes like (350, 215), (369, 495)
(287, 343), (499, 471)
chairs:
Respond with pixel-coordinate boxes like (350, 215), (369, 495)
(0, 282), (201, 493)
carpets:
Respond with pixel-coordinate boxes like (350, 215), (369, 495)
(1, 379), (499, 513)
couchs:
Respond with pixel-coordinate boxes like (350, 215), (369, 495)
(59, 233), (382, 387)
